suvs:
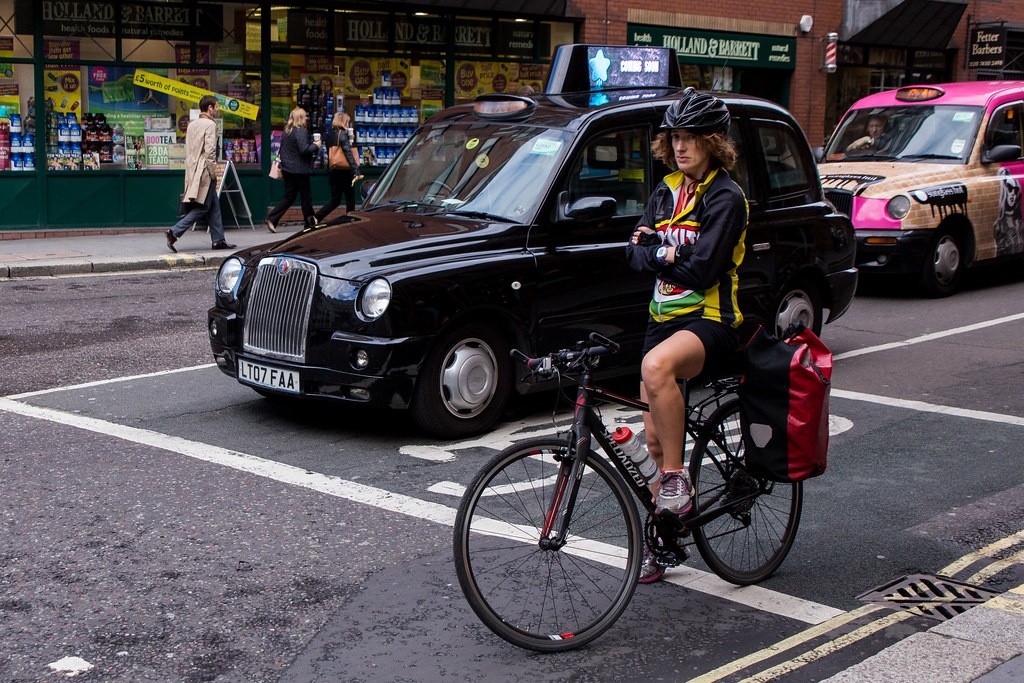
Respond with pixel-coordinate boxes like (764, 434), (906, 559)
(205, 81), (860, 443)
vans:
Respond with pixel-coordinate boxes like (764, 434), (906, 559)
(809, 77), (1024, 301)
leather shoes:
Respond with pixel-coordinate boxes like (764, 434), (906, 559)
(164, 229), (179, 253)
(212, 240), (237, 249)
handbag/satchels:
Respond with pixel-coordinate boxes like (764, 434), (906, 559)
(179, 192), (191, 216)
(269, 155), (282, 178)
(741, 322), (833, 482)
(328, 128), (360, 171)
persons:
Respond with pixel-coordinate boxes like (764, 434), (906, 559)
(307, 112), (364, 231)
(845, 114), (894, 155)
(165, 95), (237, 253)
(627, 89), (748, 584)
(263, 108), (322, 233)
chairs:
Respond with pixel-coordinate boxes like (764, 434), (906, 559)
(587, 138), (626, 171)
(759, 129), (794, 173)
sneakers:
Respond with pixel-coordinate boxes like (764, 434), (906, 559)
(638, 539), (689, 582)
(652, 468), (695, 516)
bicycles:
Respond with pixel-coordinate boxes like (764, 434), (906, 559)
(451, 330), (805, 654)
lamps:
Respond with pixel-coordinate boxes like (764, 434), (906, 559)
(820, 33), (838, 73)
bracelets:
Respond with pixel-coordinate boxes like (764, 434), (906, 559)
(867, 137), (872, 143)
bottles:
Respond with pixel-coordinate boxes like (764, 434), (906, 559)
(144, 116), (151, 131)
(0, 111), (124, 171)
(613, 426), (661, 484)
(297, 70), (424, 170)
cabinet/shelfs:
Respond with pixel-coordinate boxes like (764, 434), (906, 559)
(355, 123), (419, 166)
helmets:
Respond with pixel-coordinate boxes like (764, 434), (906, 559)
(659, 92), (731, 134)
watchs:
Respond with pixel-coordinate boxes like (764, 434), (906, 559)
(656, 245), (670, 265)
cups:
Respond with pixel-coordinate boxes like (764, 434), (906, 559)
(347, 128), (354, 137)
(313, 133), (321, 142)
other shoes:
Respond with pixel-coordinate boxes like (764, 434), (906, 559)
(307, 216), (319, 230)
(265, 219), (278, 233)
